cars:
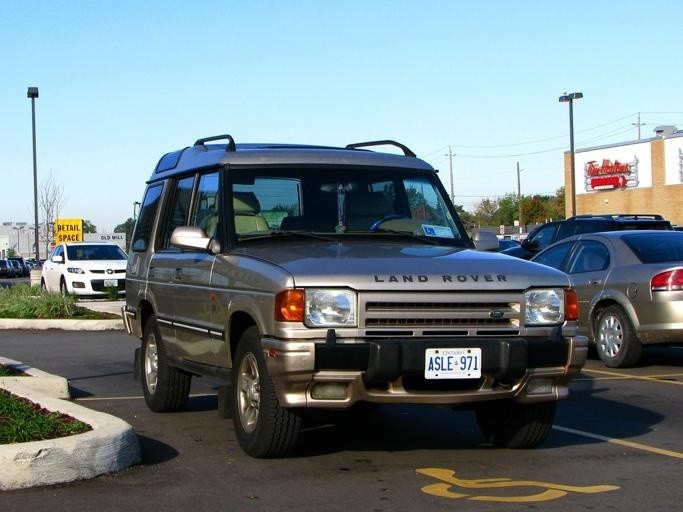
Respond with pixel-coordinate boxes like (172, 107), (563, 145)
(527, 230), (683, 370)
(485, 240), (522, 252)
(39, 242), (128, 299)
(0, 256), (37, 289)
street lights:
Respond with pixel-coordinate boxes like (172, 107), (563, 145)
(559, 93), (583, 216)
(27, 87), (40, 270)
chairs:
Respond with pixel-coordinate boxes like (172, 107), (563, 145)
(583, 249), (606, 271)
(207, 191), (392, 237)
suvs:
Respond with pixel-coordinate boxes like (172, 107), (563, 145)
(496, 212), (683, 269)
(121, 135), (589, 458)
